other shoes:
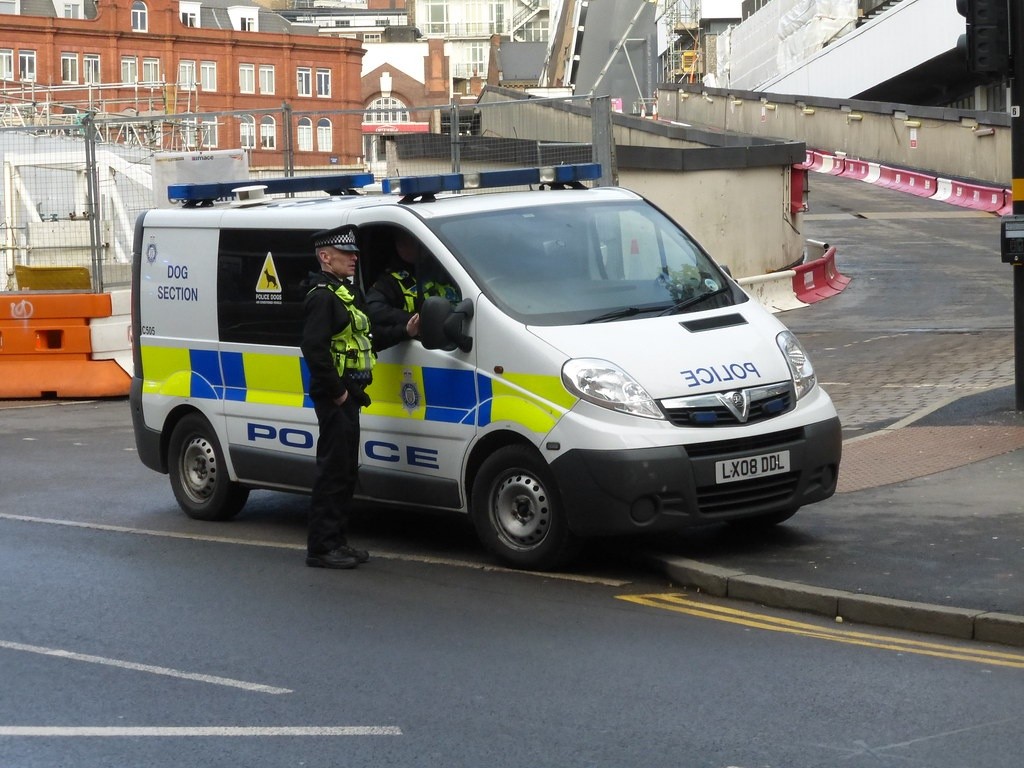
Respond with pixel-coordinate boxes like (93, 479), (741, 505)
(306, 547), (358, 569)
(337, 545), (369, 561)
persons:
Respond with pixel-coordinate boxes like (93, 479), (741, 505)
(363, 230), (460, 338)
(300, 225), (419, 571)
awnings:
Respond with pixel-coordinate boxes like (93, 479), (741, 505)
(361, 119), (429, 134)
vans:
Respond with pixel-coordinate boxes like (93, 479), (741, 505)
(129, 162), (843, 567)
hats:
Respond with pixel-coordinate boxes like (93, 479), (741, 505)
(311, 224), (362, 252)
